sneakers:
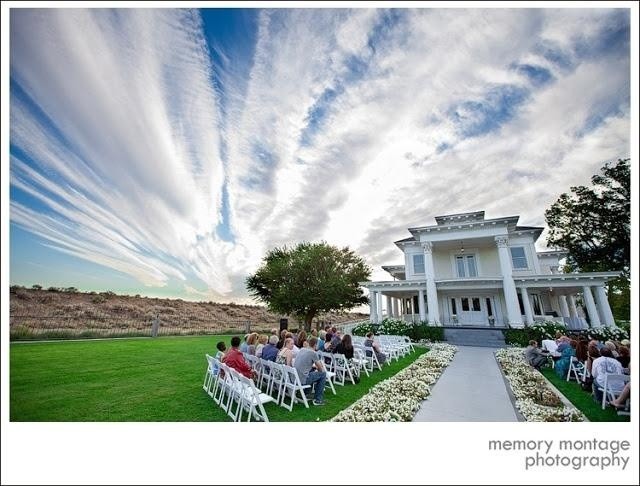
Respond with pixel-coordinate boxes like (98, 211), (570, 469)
(313, 400), (327, 406)
(610, 400), (626, 411)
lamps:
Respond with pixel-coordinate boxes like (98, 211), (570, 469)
(460, 242), (465, 253)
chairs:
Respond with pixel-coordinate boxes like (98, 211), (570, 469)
(541, 329), (631, 422)
(201, 333), (416, 422)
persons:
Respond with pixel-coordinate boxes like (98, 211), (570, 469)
(212, 325), (387, 406)
(525, 332), (630, 411)
